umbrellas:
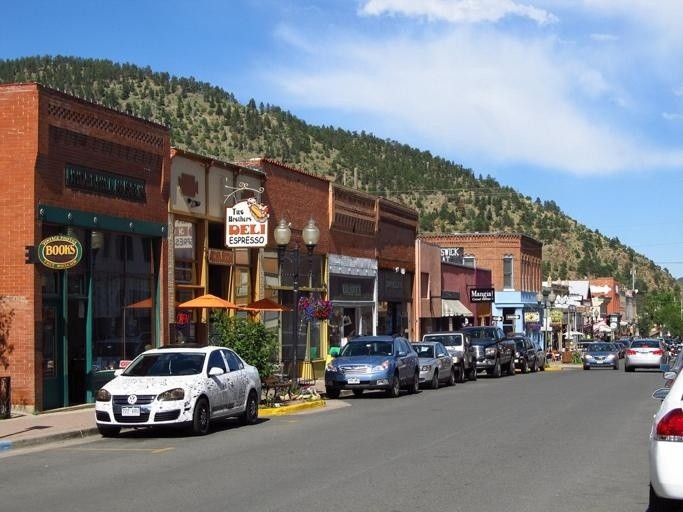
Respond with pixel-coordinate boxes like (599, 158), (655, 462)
(177, 293), (239, 311)
(124, 296), (181, 309)
(234, 298), (293, 312)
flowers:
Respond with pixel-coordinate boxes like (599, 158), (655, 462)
(297, 291), (333, 321)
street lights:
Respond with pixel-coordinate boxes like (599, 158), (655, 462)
(535, 289), (556, 348)
(587, 309), (599, 338)
(273, 214), (320, 389)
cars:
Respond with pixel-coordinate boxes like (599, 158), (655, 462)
(94, 343), (262, 436)
(325, 325), (545, 397)
(584, 339), (683, 505)
(104, 331), (183, 354)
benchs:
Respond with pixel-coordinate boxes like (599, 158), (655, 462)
(260, 384), (293, 403)
